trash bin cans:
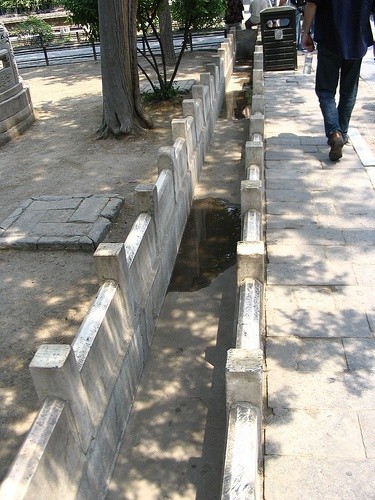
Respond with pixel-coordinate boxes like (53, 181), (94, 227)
(259, 6), (298, 71)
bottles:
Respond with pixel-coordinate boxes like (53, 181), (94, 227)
(303, 51), (313, 74)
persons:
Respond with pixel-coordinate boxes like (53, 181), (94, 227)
(271, 0), (279, 27)
(300, 0), (375, 161)
(279, 0), (302, 45)
(245, 0), (272, 30)
(222, 0), (244, 38)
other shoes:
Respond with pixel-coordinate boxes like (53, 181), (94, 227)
(328, 130), (344, 161)
(343, 133), (349, 144)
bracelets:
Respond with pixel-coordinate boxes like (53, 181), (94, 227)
(302, 32), (311, 36)
